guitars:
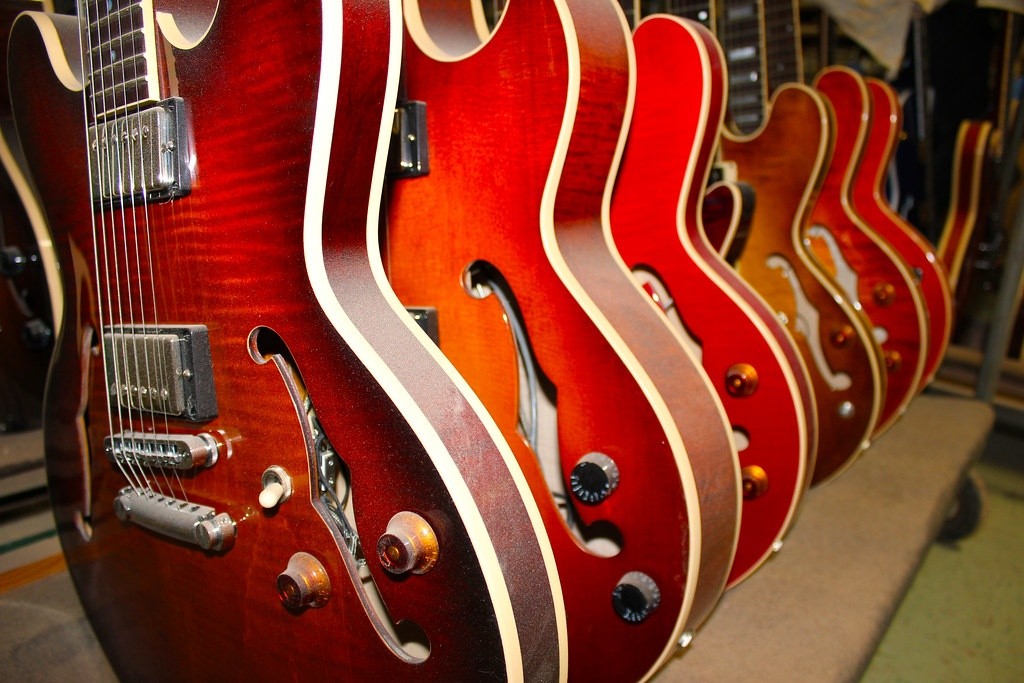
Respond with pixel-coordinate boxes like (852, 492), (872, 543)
(612, 0), (821, 596)
(392, 0), (745, 683)
(5, 0), (570, 683)
(935, 10), (1018, 345)
(666, 0), (956, 495)
